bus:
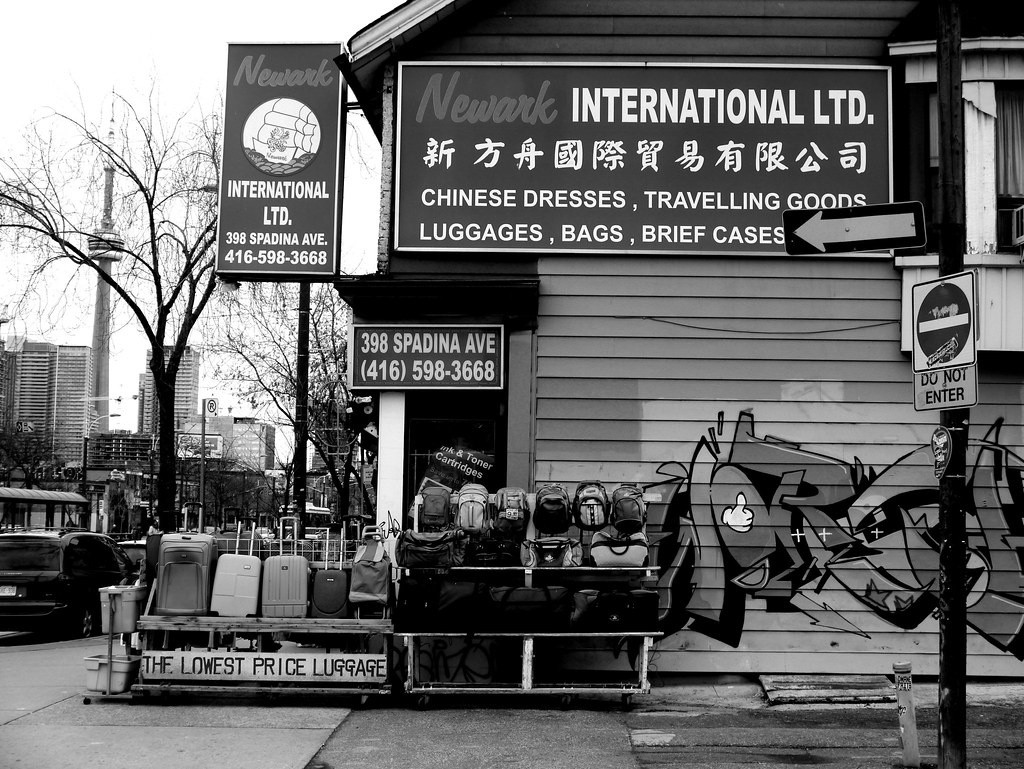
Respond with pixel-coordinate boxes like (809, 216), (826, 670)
(278, 500), (333, 539)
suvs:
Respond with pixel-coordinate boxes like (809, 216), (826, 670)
(0, 524), (141, 639)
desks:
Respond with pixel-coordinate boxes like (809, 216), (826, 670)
(135, 615), (394, 705)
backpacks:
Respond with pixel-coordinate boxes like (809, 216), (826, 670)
(610, 484), (648, 533)
(453, 483), (491, 539)
(533, 483), (572, 534)
(413, 486), (450, 533)
(494, 487), (531, 542)
(572, 480), (611, 531)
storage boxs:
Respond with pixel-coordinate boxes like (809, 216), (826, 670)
(98, 585), (147, 634)
(83, 655), (140, 693)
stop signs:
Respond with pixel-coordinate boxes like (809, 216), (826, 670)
(908, 271), (977, 374)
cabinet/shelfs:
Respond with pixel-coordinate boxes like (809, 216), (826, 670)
(393, 564), (664, 708)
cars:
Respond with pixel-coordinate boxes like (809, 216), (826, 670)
(116, 537), (147, 572)
(178, 523), (274, 539)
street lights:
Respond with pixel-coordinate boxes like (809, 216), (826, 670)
(146, 429), (186, 527)
(81, 414), (122, 528)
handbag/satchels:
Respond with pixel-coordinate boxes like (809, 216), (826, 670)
(520, 536), (583, 568)
(589, 530), (650, 567)
(416, 635), (492, 684)
(486, 579), (573, 633)
(463, 527), (523, 567)
(396, 529), (465, 567)
(571, 587), (660, 633)
(393, 577), (488, 633)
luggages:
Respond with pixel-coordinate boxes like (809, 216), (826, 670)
(210, 517), (261, 618)
(348, 526), (392, 619)
(264, 516), (311, 616)
(313, 523), (348, 618)
(154, 502), (217, 615)
(211, 506), (362, 561)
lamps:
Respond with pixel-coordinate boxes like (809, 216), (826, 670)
(364, 421), (379, 438)
